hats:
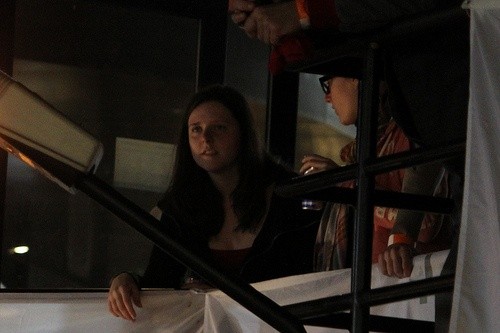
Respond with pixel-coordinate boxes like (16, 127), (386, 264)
(325, 32), (414, 82)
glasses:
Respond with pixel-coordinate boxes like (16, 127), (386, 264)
(318, 73), (339, 96)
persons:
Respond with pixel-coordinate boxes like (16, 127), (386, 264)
(376, 150), (464, 279)
(107, 85), (341, 322)
(228, 0), (470, 167)
(298, 72), (452, 272)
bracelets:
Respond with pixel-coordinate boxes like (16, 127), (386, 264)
(295, 0), (311, 30)
(384, 233), (412, 250)
